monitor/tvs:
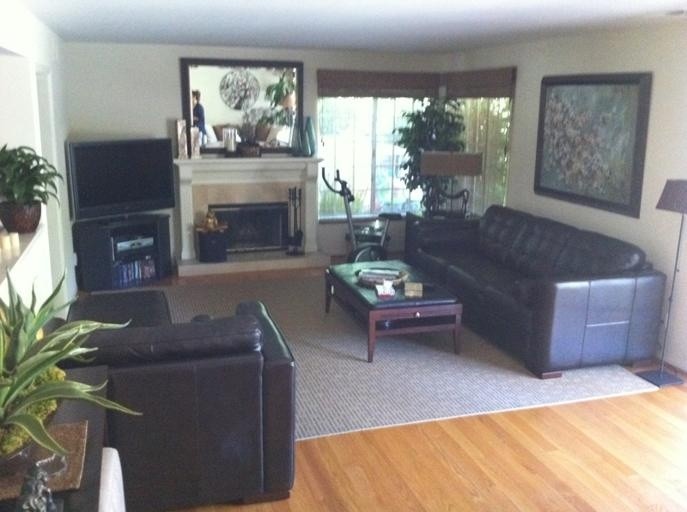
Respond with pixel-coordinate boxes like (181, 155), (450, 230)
(64, 138), (175, 226)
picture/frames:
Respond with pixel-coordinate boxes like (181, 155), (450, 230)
(532, 70), (654, 219)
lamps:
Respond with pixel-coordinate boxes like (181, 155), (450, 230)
(637, 179), (687, 387)
(418, 150), (484, 211)
(222, 123), (238, 157)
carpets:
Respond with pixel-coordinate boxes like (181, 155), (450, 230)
(161, 271), (660, 446)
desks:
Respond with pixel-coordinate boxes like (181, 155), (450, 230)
(65, 290), (171, 327)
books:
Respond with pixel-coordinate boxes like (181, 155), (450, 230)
(115, 254), (156, 284)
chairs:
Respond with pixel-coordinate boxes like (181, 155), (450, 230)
(45, 301), (299, 511)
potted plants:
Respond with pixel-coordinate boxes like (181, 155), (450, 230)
(393, 86), (467, 263)
(0, 265), (145, 470)
(263, 71), (296, 109)
(0, 144), (65, 235)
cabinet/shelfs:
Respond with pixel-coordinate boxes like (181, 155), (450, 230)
(0, 361), (110, 511)
(71, 213), (173, 293)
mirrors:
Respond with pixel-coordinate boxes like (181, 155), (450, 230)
(177, 54), (304, 155)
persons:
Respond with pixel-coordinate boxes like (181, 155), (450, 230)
(192, 90), (207, 143)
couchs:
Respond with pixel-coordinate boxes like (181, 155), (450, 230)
(410, 203), (669, 382)
